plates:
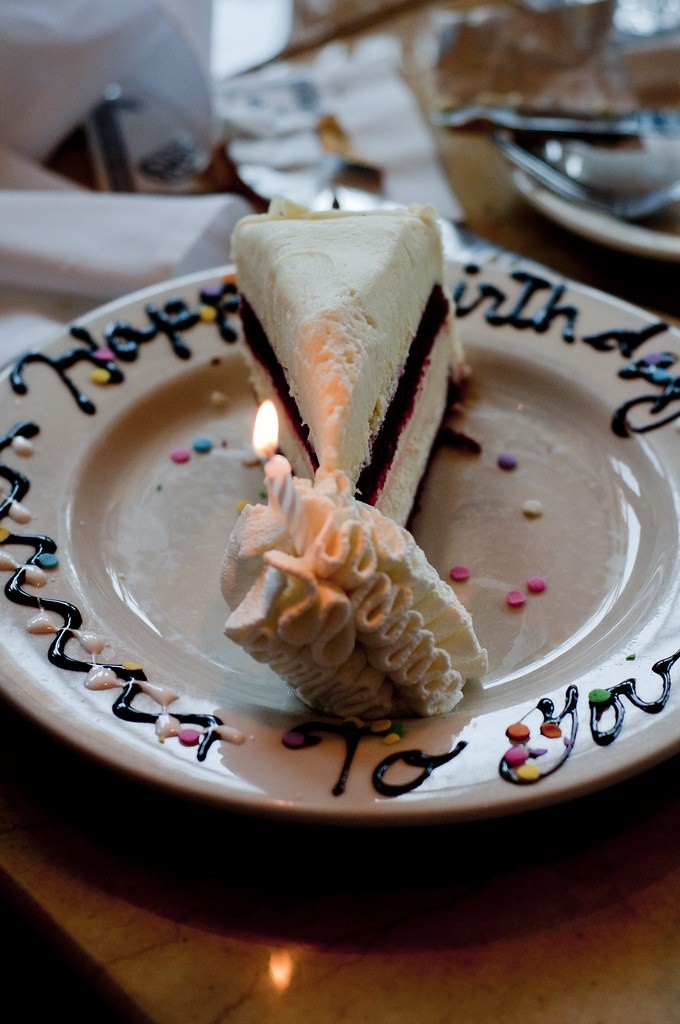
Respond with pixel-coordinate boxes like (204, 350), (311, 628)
(0, 250), (680, 827)
(507, 42), (680, 262)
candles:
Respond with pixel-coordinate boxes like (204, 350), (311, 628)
(253, 401), (308, 550)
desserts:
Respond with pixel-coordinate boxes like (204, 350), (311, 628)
(219, 194), (489, 722)
